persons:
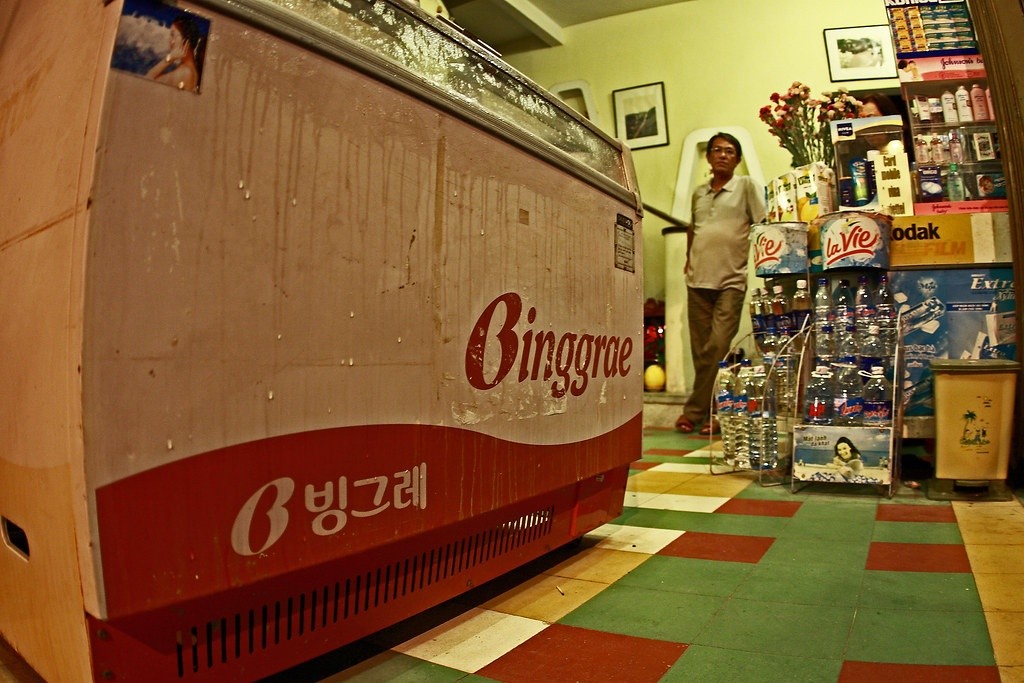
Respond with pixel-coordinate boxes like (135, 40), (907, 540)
(675, 132), (767, 435)
(826, 436), (864, 470)
(143, 16), (198, 94)
(980, 176), (994, 194)
(897, 59), (924, 82)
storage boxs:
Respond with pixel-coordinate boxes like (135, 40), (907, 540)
(765, 161), (839, 251)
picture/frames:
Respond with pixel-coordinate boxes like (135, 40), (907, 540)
(823, 23), (899, 83)
(612, 81), (669, 151)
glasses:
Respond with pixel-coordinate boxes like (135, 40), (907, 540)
(707, 148), (738, 155)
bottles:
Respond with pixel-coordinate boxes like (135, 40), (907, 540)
(866, 150), (881, 203)
(645, 359), (665, 390)
(941, 83), (995, 122)
(949, 127), (969, 163)
(901, 296), (946, 338)
(943, 136), (951, 160)
(916, 134), (928, 163)
(947, 164), (964, 200)
(930, 132), (943, 163)
(715, 359), (777, 471)
(749, 275), (895, 426)
(910, 162), (916, 170)
(946, 164), (964, 201)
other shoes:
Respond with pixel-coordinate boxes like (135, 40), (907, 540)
(675, 415), (694, 433)
(698, 419), (720, 435)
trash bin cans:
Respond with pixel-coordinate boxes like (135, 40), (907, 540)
(927, 357), (1022, 502)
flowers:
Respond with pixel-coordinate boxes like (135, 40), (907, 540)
(758, 81), (865, 172)
